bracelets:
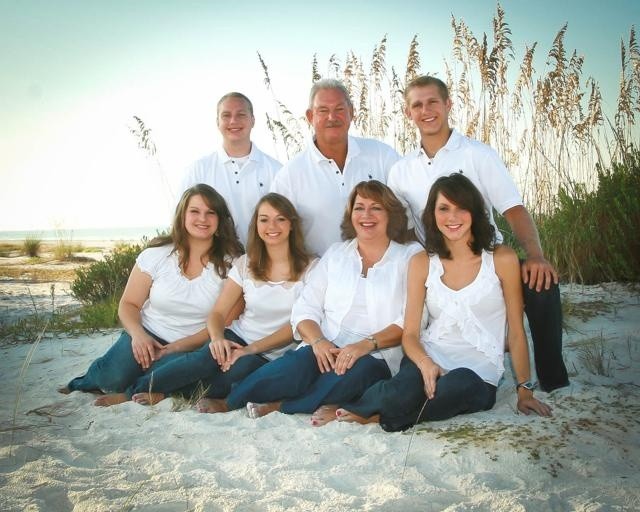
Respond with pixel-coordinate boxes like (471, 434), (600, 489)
(416, 355), (431, 368)
(310, 335), (326, 345)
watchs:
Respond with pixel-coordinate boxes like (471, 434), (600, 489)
(517, 381), (538, 389)
(367, 335), (378, 349)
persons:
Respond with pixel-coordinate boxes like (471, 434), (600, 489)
(95, 192), (327, 407)
(308, 172), (555, 433)
(386, 76), (571, 392)
(270, 79), (404, 258)
(56, 183), (247, 395)
(197, 180), (427, 416)
(186, 93), (282, 254)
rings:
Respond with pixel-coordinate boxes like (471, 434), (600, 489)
(345, 352), (354, 359)
